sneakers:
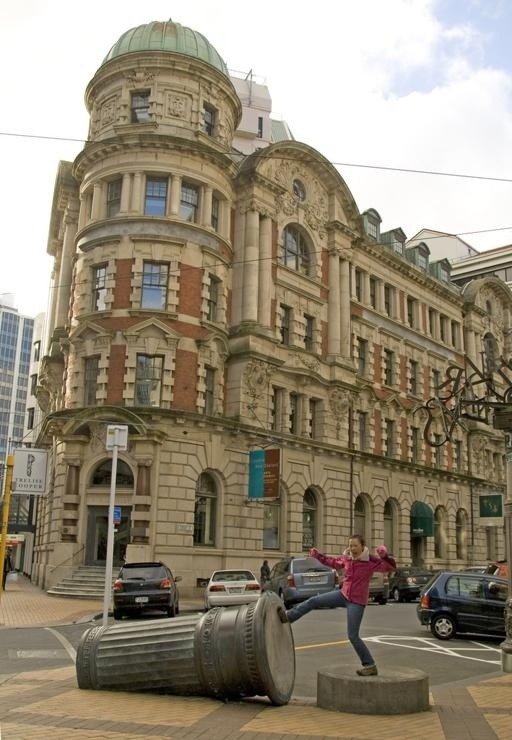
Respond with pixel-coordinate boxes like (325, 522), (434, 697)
(276, 606), (288, 623)
(356, 664), (378, 677)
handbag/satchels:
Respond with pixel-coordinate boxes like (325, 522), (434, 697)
(6, 571), (18, 584)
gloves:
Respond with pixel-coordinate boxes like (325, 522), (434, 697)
(309, 547), (319, 558)
(376, 546), (386, 558)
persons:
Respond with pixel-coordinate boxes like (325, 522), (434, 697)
(260, 560), (270, 584)
(2, 550), (12, 591)
(277, 534), (396, 677)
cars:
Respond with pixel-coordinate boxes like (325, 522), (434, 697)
(261, 558), (512, 640)
(205, 569), (261, 613)
(113, 562), (182, 620)
